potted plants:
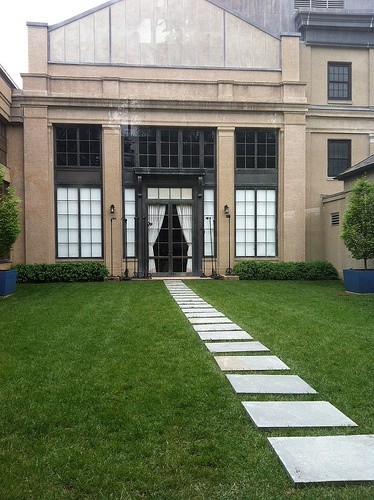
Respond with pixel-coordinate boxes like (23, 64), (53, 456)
(0, 166), (23, 298)
(339, 175), (374, 294)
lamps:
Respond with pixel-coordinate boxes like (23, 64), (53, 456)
(224, 204), (231, 218)
(110, 204), (115, 213)
